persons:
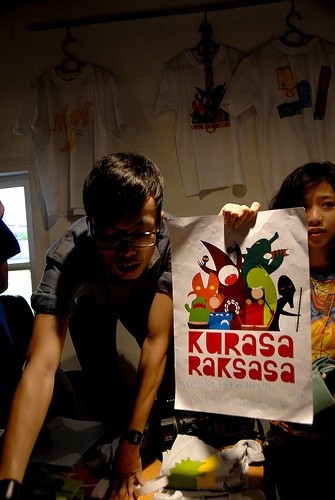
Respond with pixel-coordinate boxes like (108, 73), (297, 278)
(0, 150), (256, 499)
(218, 162), (335, 500)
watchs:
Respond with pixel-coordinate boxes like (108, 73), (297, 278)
(122, 428), (144, 445)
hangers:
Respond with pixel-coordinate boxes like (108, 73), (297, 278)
(280, 11), (313, 47)
(191, 21), (220, 57)
(56, 38), (85, 73)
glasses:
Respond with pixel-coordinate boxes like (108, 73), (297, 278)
(89, 219), (160, 247)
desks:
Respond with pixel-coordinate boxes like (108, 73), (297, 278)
(123, 438), (264, 500)
